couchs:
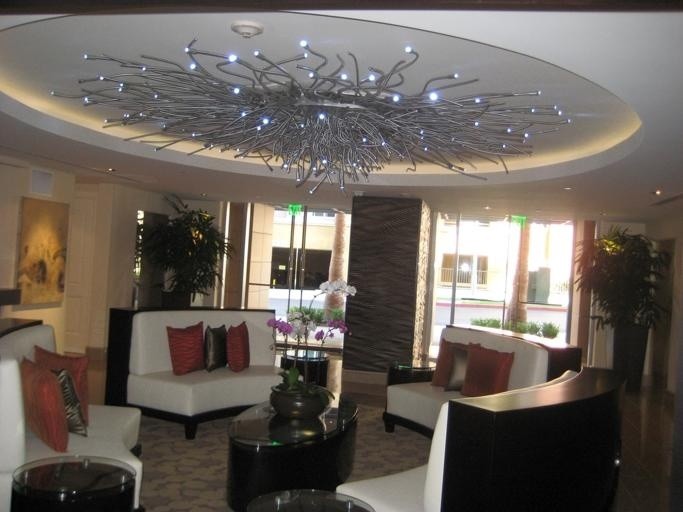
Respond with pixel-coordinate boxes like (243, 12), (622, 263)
(335, 366), (621, 511)
(384, 326), (582, 442)
(1, 318), (146, 512)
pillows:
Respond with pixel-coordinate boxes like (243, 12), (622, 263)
(104, 306), (303, 439)
(445, 349), (471, 391)
(34, 345), (89, 425)
(227, 322), (250, 371)
(52, 369), (88, 436)
(431, 339), (468, 388)
(168, 322), (205, 375)
(205, 324), (227, 372)
(460, 348), (514, 398)
(21, 357), (68, 452)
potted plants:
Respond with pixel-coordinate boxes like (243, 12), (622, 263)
(132, 194), (234, 312)
(573, 227), (668, 391)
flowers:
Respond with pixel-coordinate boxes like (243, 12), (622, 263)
(267, 280), (356, 392)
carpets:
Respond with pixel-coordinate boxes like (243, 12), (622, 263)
(131, 403), (432, 512)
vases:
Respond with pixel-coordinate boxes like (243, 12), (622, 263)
(270, 383), (332, 419)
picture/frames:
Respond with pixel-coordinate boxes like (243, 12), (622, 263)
(12, 197), (71, 306)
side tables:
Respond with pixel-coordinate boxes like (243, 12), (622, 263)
(248, 489), (376, 512)
(9, 455), (136, 512)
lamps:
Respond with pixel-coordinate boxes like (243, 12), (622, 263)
(49, 38), (570, 195)
